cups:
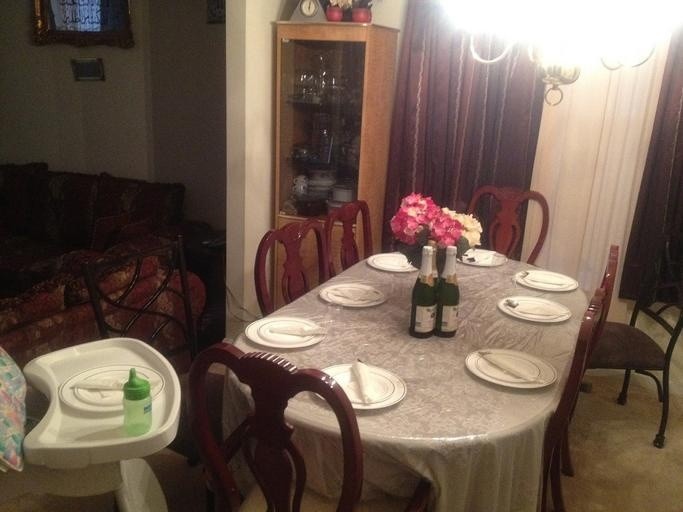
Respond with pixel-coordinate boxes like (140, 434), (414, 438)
(409, 241), (460, 339)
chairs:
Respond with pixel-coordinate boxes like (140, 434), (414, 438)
(254, 218), (331, 317)
(0, 337), (182, 512)
(187, 344), (430, 512)
(325, 199), (373, 279)
(82, 235), (203, 467)
(465, 185), (549, 264)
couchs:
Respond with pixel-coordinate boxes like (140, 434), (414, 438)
(0, 163), (207, 371)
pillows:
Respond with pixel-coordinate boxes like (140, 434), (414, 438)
(29, 171), (101, 249)
(92, 173), (184, 253)
(0, 163), (49, 236)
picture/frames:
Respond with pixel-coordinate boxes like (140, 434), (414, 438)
(32, 1), (134, 48)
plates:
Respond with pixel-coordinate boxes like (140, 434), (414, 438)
(465, 348), (558, 390)
(319, 249), (579, 324)
(58, 363), (166, 412)
(74, 370), (149, 406)
(258, 319), (316, 343)
(312, 363), (406, 410)
(244, 317), (325, 348)
(328, 369), (395, 402)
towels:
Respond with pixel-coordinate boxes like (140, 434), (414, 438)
(352, 356), (376, 404)
(478, 350), (544, 383)
(270, 325), (328, 336)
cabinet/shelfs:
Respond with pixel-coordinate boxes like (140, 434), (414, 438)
(270, 21), (400, 313)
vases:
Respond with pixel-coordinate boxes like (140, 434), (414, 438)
(326, 4), (343, 22)
(352, 0), (372, 24)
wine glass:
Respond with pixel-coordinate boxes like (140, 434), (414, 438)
(290, 54), (342, 98)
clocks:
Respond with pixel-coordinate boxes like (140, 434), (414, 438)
(289, 0), (328, 21)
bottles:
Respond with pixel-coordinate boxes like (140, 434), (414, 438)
(120, 367), (152, 436)
(280, 114), (362, 230)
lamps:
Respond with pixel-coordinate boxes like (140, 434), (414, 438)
(433, 1), (682, 105)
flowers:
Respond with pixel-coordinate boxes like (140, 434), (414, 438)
(390, 192), (483, 276)
(329, 0), (352, 11)
